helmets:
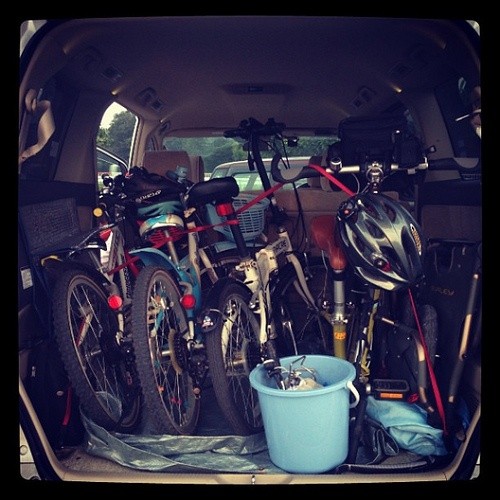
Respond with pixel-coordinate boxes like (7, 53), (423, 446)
(132, 177), (184, 221)
(334, 194), (426, 292)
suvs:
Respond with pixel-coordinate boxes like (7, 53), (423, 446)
(207, 155), (314, 191)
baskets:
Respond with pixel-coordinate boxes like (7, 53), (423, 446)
(222, 191), (271, 238)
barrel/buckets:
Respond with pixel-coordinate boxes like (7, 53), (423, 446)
(249, 352), (361, 475)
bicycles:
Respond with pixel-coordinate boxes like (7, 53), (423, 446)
(37, 113), (438, 473)
